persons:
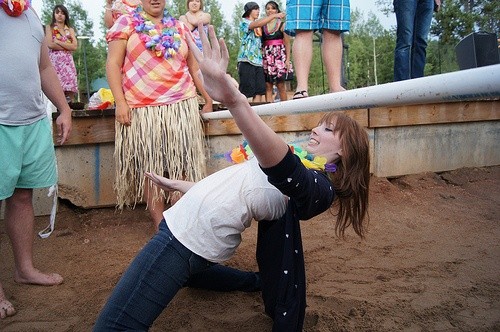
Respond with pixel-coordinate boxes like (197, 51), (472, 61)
(260, 1), (293, 102)
(91, 21), (372, 332)
(390, 0), (441, 82)
(283, 0), (351, 98)
(105, 1), (213, 232)
(0, 0), (74, 319)
(45, 5), (79, 106)
(237, 2), (287, 103)
(178, 0), (211, 54)
(105, 1), (144, 29)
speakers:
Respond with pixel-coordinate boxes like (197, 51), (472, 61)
(455, 30), (500, 69)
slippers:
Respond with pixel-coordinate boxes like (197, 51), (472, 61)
(293, 90), (308, 100)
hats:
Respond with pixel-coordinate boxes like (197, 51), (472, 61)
(242, 1), (257, 18)
